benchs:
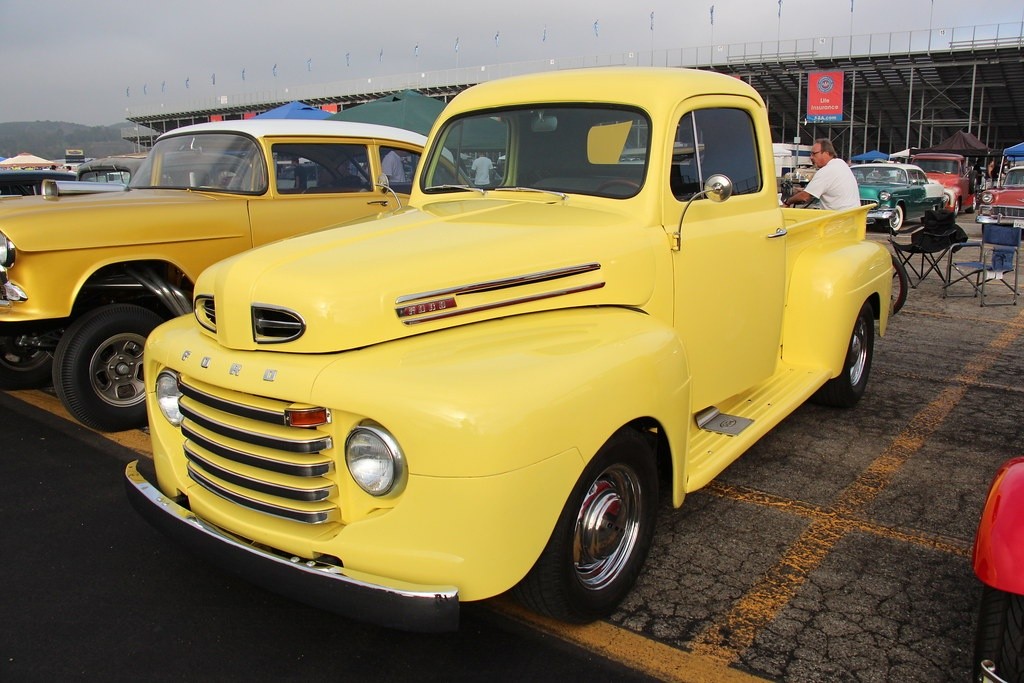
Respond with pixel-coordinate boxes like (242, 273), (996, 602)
(302, 186), (368, 192)
(533, 173), (648, 197)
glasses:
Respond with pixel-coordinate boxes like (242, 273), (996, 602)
(809, 150), (821, 156)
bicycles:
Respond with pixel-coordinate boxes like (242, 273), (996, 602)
(789, 196), (908, 317)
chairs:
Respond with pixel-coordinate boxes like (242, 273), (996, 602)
(943, 225), (1021, 308)
(887, 211), (968, 287)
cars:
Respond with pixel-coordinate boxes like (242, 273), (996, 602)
(120, 65), (894, 641)
(848, 162), (944, 233)
(910, 153), (977, 217)
(967, 453), (1024, 683)
(976, 165), (1024, 241)
(0, 119), (473, 433)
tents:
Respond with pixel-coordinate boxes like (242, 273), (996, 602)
(850, 130), (995, 197)
(0, 153), (61, 166)
(246, 101), (333, 119)
(997, 142), (1024, 188)
(325, 90), (512, 152)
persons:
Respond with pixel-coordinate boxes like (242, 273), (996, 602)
(382, 146), (410, 182)
(470, 151), (494, 188)
(783, 138), (861, 209)
(49, 165), (57, 171)
(66, 166), (75, 173)
(986, 160), (1000, 188)
(9, 165), (17, 171)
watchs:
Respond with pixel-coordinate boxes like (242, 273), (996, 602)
(784, 199), (788, 205)
